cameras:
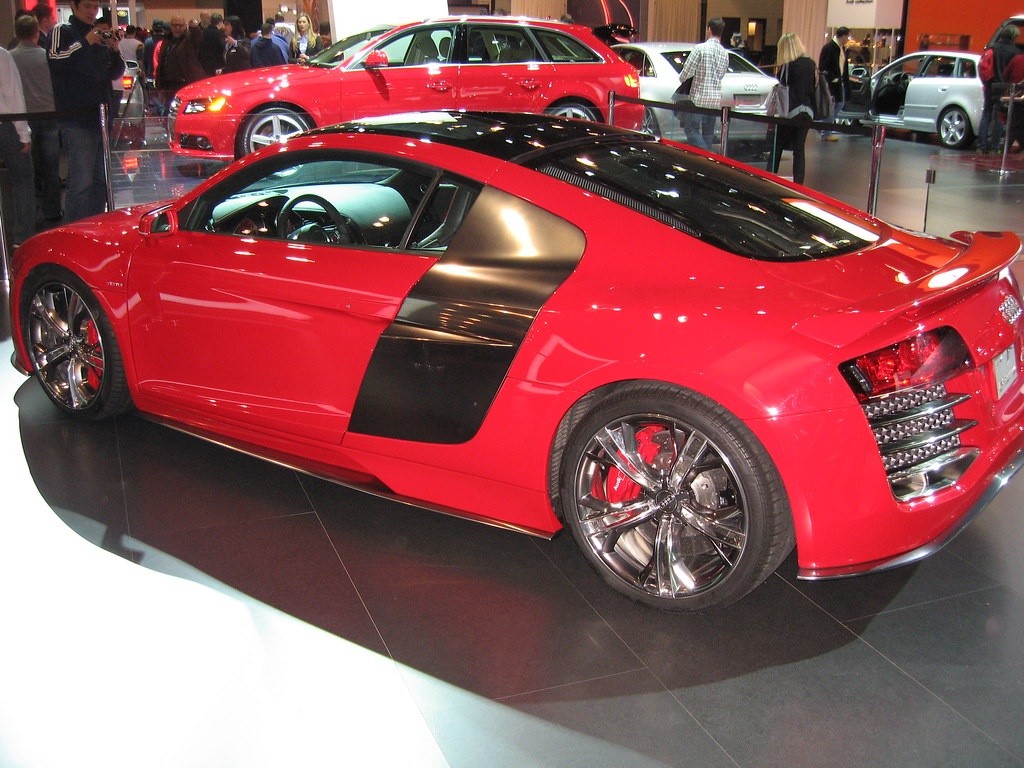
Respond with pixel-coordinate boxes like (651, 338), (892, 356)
(97, 30), (113, 46)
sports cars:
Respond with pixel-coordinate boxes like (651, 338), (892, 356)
(6, 116), (1024, 613)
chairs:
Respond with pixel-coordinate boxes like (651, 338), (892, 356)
(421, 37), (440, 64)
(899, 63), (954, 109)
(490, 48), (533, 63)
(409, 187), (478, 249)
(439, 37), (451, 63)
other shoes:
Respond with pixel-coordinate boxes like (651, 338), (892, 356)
(820, 134), (839, 141)
(157, 106), (164, 117)
(58, 176), (66, 190)
(45, 210), (64, 223)
(975, 148), (986, 155)
(992, 148), (1003, 155)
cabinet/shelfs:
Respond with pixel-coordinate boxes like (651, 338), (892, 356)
(832, 27), (896, 77)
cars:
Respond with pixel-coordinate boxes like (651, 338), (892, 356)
(607, 41), (780, 145)
(832, 51), (1003, 151)
(113, 60), (147, 145)
(168, 14), (645, 161)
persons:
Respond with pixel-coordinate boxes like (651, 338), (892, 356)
(0, 0), (60, 258)
(46, 0), (126, 225)
(678, 16), (729, 150)
(766, 32), (819, 186)
(977, 24), (1024, 155)
(819, 26), (850, 142)
(95, 10), (332, 117)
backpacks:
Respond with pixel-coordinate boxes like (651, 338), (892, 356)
(978, 45), (994, 83)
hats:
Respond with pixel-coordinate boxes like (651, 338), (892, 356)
(153, 19), (163, 29)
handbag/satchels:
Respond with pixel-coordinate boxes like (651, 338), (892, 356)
(766, 63), (789, 119)
(813, 67), (835, 120)
(159, 64), (189, 90)
